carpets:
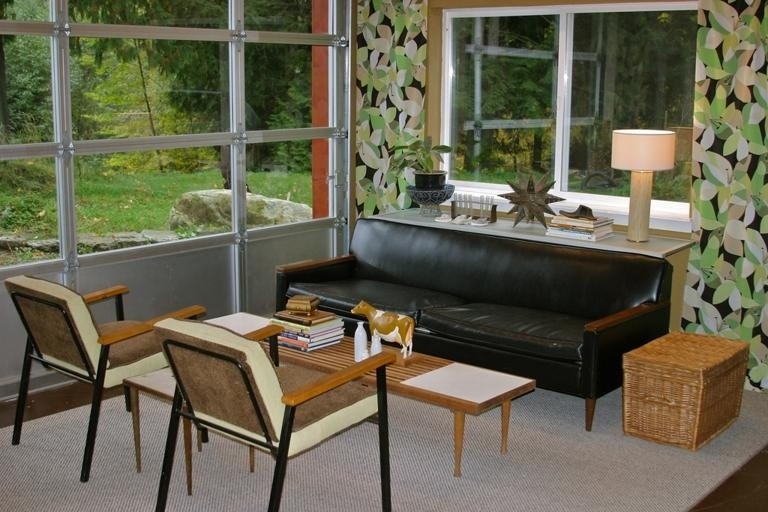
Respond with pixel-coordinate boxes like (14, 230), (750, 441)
(0, 364), (767, 512)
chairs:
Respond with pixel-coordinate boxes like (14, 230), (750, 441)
(2, 271), (205, 485)
(155, 314), (398, 512)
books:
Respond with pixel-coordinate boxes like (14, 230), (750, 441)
(545, 214), (615, 243)
(265, 308), (345, 352)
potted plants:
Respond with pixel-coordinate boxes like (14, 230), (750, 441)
(393, 134), (454, 218)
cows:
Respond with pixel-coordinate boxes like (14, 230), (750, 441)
(351, 300), (415, 359)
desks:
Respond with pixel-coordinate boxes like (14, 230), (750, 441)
(380, 206), (696, 336)
(120, 368), (256, 500)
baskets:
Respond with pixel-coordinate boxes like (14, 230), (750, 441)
(622, 330), (748, 452)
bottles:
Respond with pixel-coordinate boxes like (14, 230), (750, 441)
(354, 321), (368, 363)
(370, 336), (383, 356)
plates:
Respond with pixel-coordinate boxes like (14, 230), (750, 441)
(434, 216), (489, 227)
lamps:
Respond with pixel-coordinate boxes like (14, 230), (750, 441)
(609, 127), (676, 244)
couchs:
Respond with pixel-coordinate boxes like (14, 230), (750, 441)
(274, 218), (672, 434)
